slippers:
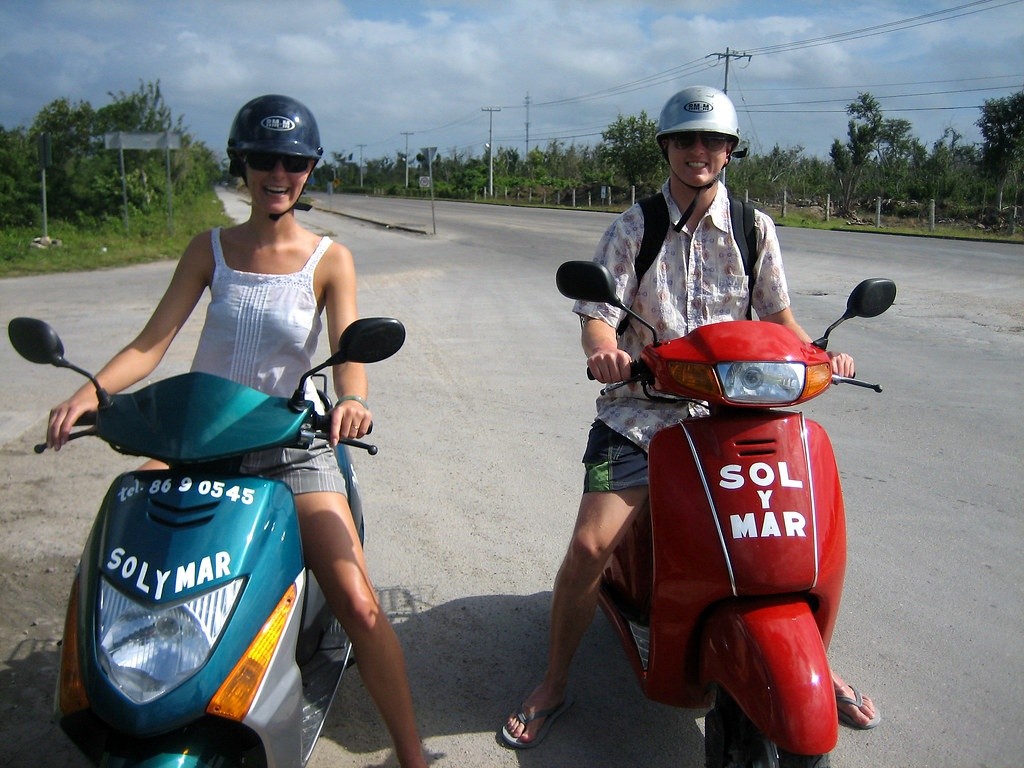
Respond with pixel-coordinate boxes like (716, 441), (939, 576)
(502, 685), (574, 748)
(836, 684), (882, 729)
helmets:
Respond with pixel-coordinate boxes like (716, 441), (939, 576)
(226, 95), (323, 161)
(655, 85), (740, 151)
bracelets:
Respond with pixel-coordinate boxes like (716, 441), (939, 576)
(335, 395), (368, 411)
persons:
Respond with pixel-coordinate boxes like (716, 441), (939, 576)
(502, 86), (882, 749)
(48, 93), (425, 768)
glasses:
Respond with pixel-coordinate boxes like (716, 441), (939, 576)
(665, 131), (729, 151)
(243, 149), (316, 173)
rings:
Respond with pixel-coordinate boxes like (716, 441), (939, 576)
(352, 425), (360, 429)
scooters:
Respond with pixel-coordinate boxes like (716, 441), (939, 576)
(554, 259), (897, 768)
(6, 313), (411, 768)
